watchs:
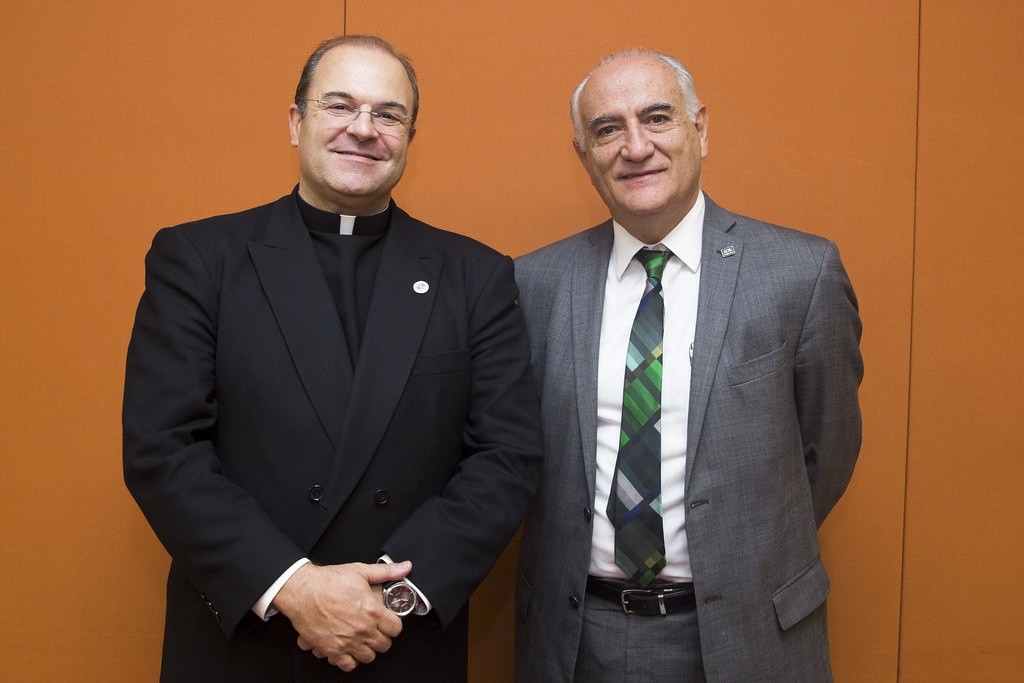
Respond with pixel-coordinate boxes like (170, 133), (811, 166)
(382, 581), (418, 617)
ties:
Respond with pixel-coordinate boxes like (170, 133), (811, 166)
(607, 249), (674, 590)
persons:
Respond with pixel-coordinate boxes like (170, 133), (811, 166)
(512, 47), (864, 683)
(122, 35), (543, 683)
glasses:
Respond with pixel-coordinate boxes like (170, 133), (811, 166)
(305, 96), (415, 136)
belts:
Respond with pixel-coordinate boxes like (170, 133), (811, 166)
(585, 573), (696, 615)
